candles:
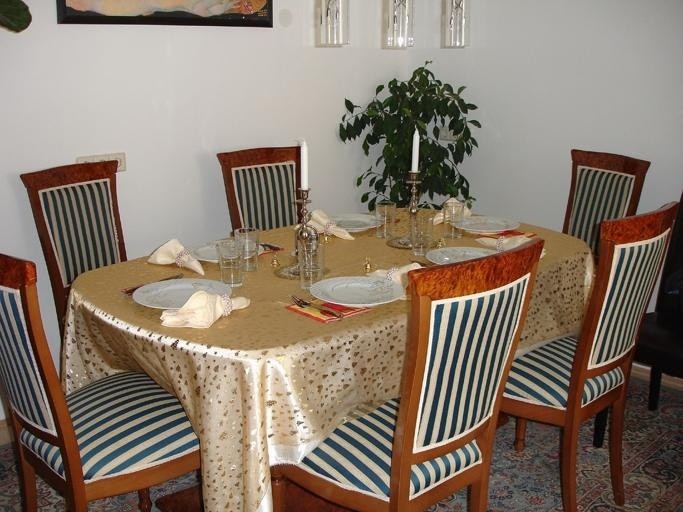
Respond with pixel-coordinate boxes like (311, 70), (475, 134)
(299, 137), (309, 190)
(410, 125), (420, 172)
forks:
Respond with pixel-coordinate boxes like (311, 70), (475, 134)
(286, 294), (341, 316)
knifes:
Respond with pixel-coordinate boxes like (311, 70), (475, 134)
(122, 273), (185, 294)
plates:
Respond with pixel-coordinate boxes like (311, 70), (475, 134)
(192, 239), (264, 262)
(131, 276), (232, 309)
(426, 246), (501, 268)
(315, 213), (382, 236)
(308, 274), (403, 308)
(449, 215), (522, 236)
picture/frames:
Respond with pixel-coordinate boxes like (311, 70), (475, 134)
(55, 0), (274, 29)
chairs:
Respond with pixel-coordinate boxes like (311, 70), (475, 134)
(17, 157), (135, 346)
(485, 202), (677, 508)
(592, 194), (681, 449)
(213, 143), (304, 233)
(559, 150), (651, 281)
(271, 237), (544, 509)
(0, 253), (208, 511)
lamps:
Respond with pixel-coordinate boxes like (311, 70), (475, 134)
(381, 0), (414, 49)
(439, 0), (468, 49)
(317, 0), (351, 48)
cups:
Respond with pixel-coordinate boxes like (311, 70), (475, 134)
(232, 227), (260, 274)
(214, 238), (245, 289)
(297, 241), (326, 288)
(375, 201), (465, 256)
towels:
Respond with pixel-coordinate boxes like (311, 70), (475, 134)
(474, 232), (546, 263)
(292, 208), (353, 241)
(154, 285), (253, 331)
(431, 196), (470, 227)
(147, 236), (203, 276)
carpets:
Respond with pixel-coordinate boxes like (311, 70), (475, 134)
(0, 373), (681, 512)
